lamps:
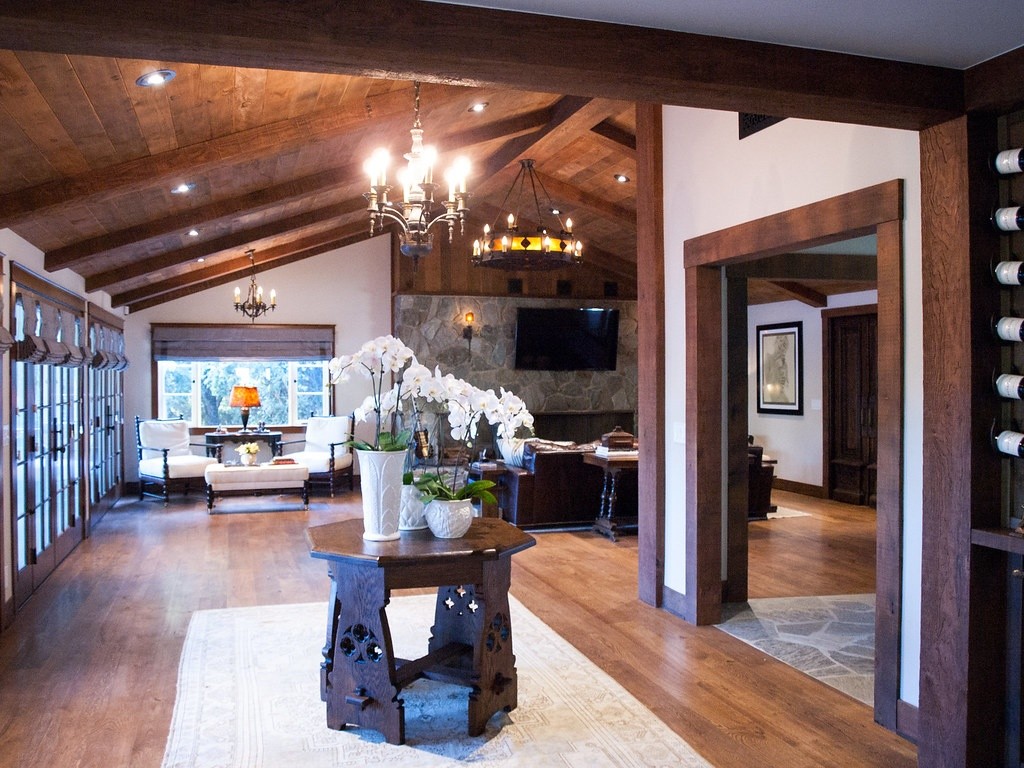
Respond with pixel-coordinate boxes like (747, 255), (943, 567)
(366, 79), (471, 258)
(463, 311), (475, 339)
(232, 250), (278, 321)
(471, 159), (582, 271)
(228, 386), (261, 431)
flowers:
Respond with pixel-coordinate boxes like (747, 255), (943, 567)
(410, 373), (535, 503)
(235, 441), (259, 456)
(400, 356), (453, 487)
(329, 334), (424, 452)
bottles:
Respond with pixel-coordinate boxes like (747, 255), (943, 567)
(992, 261), (1024, 286)
(988, 148), (1024, 174)
(995, 373), (1024, 400)
(994, 316), (1024, 342)
(993, 429), (1024, 458)
(988, 207), (1024, 230)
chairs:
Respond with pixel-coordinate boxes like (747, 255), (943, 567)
(135, 413), (222, 507)
(274, 414), (355, 499)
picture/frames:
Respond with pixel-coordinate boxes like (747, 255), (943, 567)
(755, 320), (804, 415)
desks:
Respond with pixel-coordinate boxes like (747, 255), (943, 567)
(294, 520), (536, 744)
(204, 432), (283, 465)
(204, 465), (311, 514)
(577, 449), (755, 542)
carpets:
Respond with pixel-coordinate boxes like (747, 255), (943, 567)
(161, 592), (713, 768)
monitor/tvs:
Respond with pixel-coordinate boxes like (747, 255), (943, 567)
(514, 307), (620, 372)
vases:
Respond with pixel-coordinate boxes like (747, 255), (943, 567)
(241, 452), (257, 467)
(399, 480), (431, 529)
(350, 448), (407, 542)
(426, 496), (476, 541)
